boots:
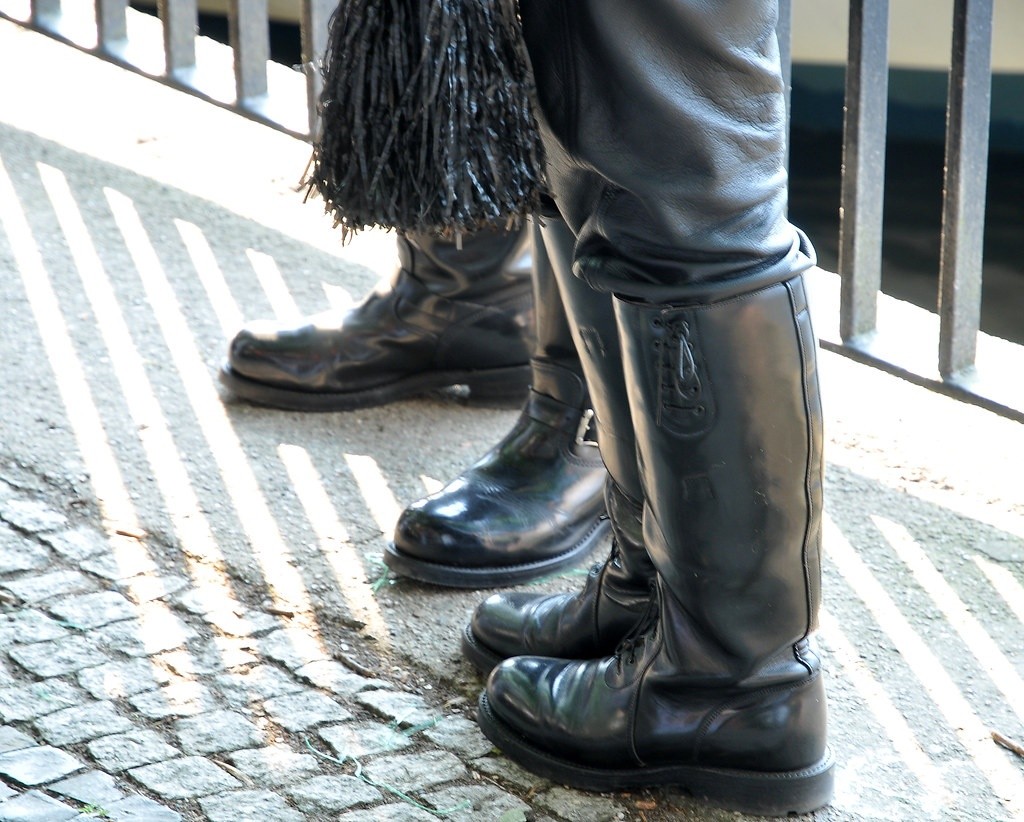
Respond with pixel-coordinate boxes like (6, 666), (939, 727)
(218, 217), (538, 412)
(478, 271), (836, 818)
(383, 213), (613, 588)
(461, 216), (659, 674)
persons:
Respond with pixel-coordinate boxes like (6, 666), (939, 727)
(213, 0), (613, 591)
(458, 0), (836, 818)
